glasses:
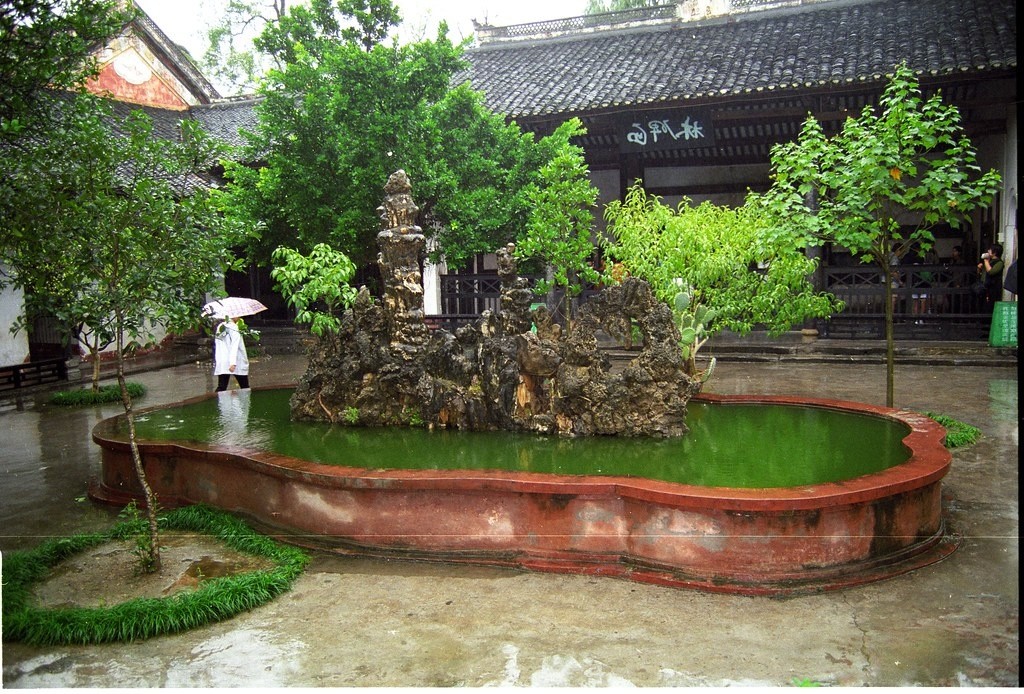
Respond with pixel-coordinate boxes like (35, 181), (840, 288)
(988, 250), (992, 253)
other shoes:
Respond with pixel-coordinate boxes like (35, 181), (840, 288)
(915, 320), (924, 324)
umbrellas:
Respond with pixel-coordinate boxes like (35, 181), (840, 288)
(201, 297), (268, 319)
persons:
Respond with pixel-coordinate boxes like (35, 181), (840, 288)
(948, 246), (963, 299)
(882, 243), (904, 313)
(959, 240), (983, 314)
(977, 244), (1005, 315)
(899, 239), (940, 314)
(213, 318), (250, 392)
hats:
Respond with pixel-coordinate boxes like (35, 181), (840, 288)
(891, 243), (904, 252)
